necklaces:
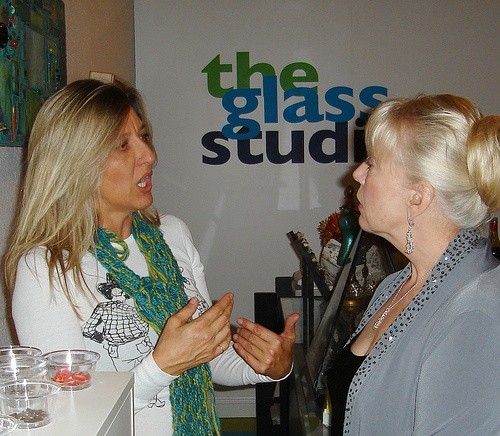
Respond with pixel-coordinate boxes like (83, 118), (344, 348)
(372, 273), (428, 329)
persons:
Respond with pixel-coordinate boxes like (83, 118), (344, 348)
(323, 90), (499, 436)
(0, 78), (303, 436)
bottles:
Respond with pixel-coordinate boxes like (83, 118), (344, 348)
(489, 217), (500, 261)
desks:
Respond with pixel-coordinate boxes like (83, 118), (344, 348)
(280, 296), (331, 436)
(0, 371), (135, 436)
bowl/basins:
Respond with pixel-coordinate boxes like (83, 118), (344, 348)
(0, 346), (101, 436)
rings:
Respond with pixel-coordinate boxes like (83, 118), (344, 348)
(218, 344), (225, 353)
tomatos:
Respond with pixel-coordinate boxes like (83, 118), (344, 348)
(52, 370), (91, 386)
